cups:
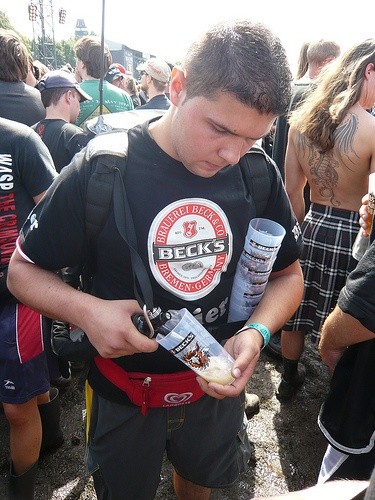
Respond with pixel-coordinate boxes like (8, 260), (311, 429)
(155, 308), (237, 386)
(228, 218), (286, 323)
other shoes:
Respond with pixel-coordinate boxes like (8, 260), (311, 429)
(278, 364), (307, 399)
(267, 334), (282, 357)
(245, 393), (258, 413)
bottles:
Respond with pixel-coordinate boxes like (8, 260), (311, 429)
(351, 173), (375, 261)
(80, 310), (172, 355)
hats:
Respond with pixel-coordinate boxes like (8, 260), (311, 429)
(38, 71), (92, 102)
(105, 67), (124, 82)
(136, 58), (171, 83)
(109, 63), (131, 75)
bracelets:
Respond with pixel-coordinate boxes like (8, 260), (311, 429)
(235, 323), (270, 351)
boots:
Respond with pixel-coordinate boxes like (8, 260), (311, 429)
(8, 460), (37, 500)
(38, 387), (66, 456)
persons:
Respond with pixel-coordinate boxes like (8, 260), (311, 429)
(0, 32), (176, 500)
(261, 38), (375, 500)
(6, 19), (303, 500)
